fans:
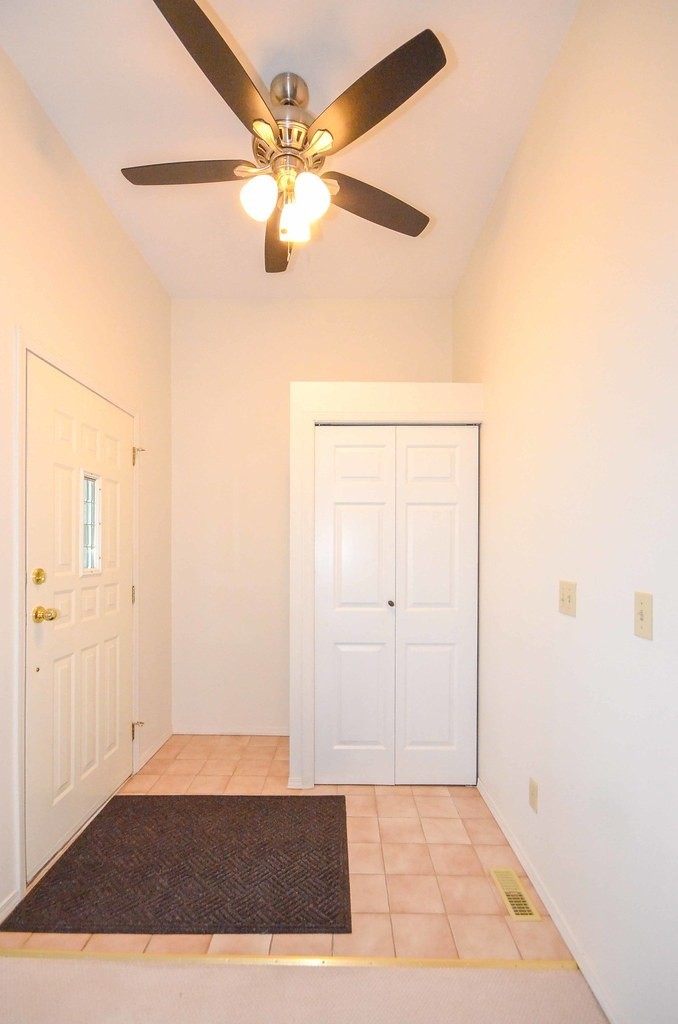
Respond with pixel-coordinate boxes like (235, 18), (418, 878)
(120, 0), (446, 273)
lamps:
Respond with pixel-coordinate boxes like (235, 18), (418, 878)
(233, 119), (340, 242)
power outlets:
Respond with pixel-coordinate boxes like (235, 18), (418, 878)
(559, 580), (577, 616)
(530, 777), (538, 813)
(634, 591), (653, 640)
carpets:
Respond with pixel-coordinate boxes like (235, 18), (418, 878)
(1, 794), (352, 934)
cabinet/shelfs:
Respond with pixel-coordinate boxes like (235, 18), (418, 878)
(314, 426), (479, 786)
